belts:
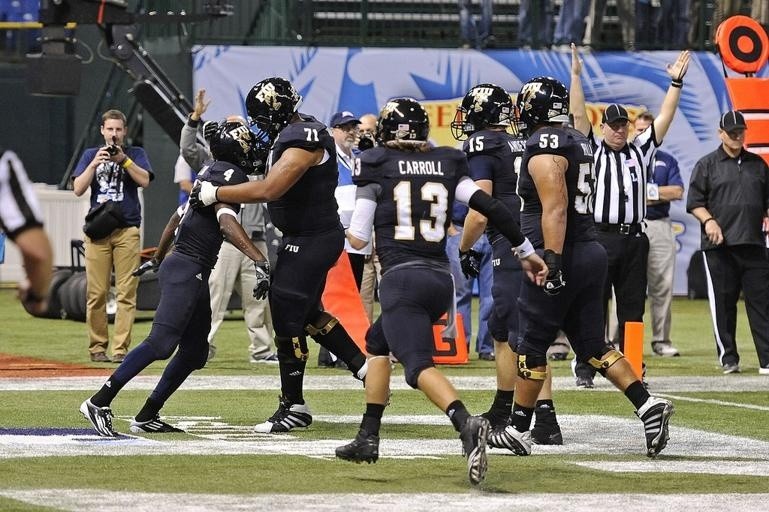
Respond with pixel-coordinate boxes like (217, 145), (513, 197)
(612, 223), (645, 234)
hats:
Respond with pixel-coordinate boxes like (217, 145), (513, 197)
(720, 111), (747, 132)
(330, 111), (363, 129)
(601, 104), (631, 124)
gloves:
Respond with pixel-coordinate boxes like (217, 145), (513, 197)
(457, 248), (482, 278)
(541, 249), (565, 295)
(252, 261), (271, 301)
(132, 255), (162, 276)
(189, 180), (218, 207)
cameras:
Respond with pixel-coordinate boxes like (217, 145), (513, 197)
(102, 135), (122, 157)
(358, 137), (372, 151)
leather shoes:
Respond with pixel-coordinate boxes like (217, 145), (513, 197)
(90, 351), (111, 362)
(112, 354), (126, 362)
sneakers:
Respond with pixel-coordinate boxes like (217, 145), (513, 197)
(254, 395), (312, 433)
(487, 414), (532, 456)
(129, 413), (186, 434)
(723, 362), (741, 374)
(634, 396), (675, 458)
(651, 343), (680, 357)
(79, 392), (119, 437)
(576, 375), (594, 388)
(460, 416), (489, 486)
(335, 429), (380, 464)
(759, 364), (769, 374)
(531, 420), (563, 445)
(250, 349), (280, 363)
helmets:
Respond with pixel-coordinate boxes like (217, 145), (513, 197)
(451, 84), (516, 141)
(373, 96), (430, 146)
(245, 77), (303, 140)
(203, 118), (268, 175)
(517, 76), (570, 137)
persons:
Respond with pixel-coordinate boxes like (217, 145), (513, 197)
(0, 148), (52, 316)
(180, 87), (281, 365)
(348, 113), (378, 161)
(487, 77), (676, 460)
(338, 96), (550, 484)
(568, 41), (691, 387)
(441, 143), (494, 362)
(78, 115), (269, 435)
(191, 77), (392, 435)
(686, 110), (769, 376)
(69, 108), (155, 363)
(453, 82), (563, 445)
(319, 112), (373, 370)
(610, 112), (684, 356)
